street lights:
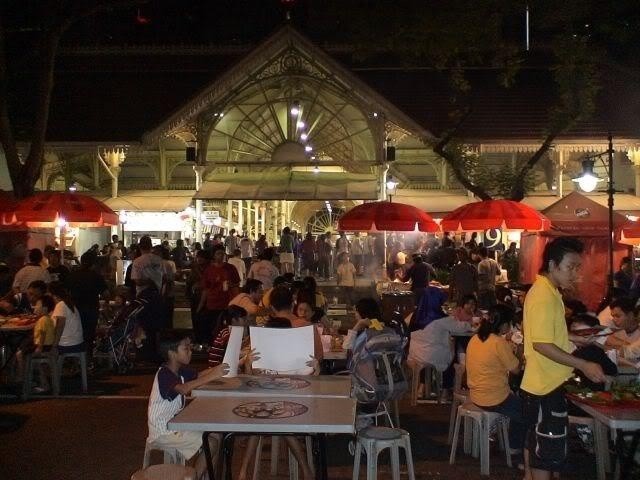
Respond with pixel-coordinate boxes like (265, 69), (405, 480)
(386, 174), (399, 203)
(572, 133), (616, 302)
(119, 210), (130, 248)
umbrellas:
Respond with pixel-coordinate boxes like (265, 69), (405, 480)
(338, 201), (440, 277)
(0, 190), (119, 265)
(439, 200), (551, 263)
(0, 191), (24, 228)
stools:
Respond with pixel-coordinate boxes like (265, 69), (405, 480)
(352, 426), (415, 480)
(143, 437), (185, 470)
(450, 404), (513, 476)
(357, 400), (395, 429)
(131, 463), (198, 480)
(406, 360), (441, 409)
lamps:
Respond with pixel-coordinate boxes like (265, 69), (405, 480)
(311, 154), (316, 159)
(306, 143), (312, 153)
(299, 120), (304, 128)
(291, 100), (298, 115)
(385, 174), (399, 189)
(572, 128), (624, 298)
(300, 131), (306, 139)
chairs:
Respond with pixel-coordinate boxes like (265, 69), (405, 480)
(122, 299), (149, 370)
(92, 300), (143, 374)
(448, 390), (472, 455)
(57, 302), (100, 393)
(22, 316), (66, 400)
(331, 320), (341, 333)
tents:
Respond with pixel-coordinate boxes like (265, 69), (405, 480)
(520, 189), (640, 314)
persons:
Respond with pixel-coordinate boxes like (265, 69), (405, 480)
(317, 234), (332, 281)
(45, 248), (68, 281)
(236, 317), (320, 479)
(152, 233), (202, 279)
(596, 299), (640, 369)
(268, 286), (324, 365)
(351, 231), (364, 275)
(50, 283), (84, 354)
(229, 278), (263, 317)
(397, 254), (437, 288)
(564, 298), (616, 391)
(408, 286), (472, 407)
(210, 305), (248, 367)
(451, 248), (478, 300)
(227, 250), (245, 289)
(341, 300), (383, 348)
(204, 228), (269, 266)
(248, 248), (279, 288)
(301, 232), (316, 276)
(196, 243), (240, 351)
(365, 231), (405, 276)
(336, 232), (349, 253)
(99, 289), (129, 325)
(337, 251), (357, 296)
(148, 331), (223, 479)
(0, 280), (48, 315)
(262, 274), (329, 322)
(518, 236), (610, 480)
(502, 243), (519, 289)
(14, 296), (54, 384)
(414, 232), (486, 267)
(476, 248), (501, 301)
(13, 248), (51, 290)
(279, 227), (294, 274)
(81, 235), (138, 286)
(612, 255), (634, 292)
(131, 236), (169, 298)
(466, 304), (524, 465)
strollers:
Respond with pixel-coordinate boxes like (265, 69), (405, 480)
(88, 297), (152, 374)
(335, 324), (411, 459)
